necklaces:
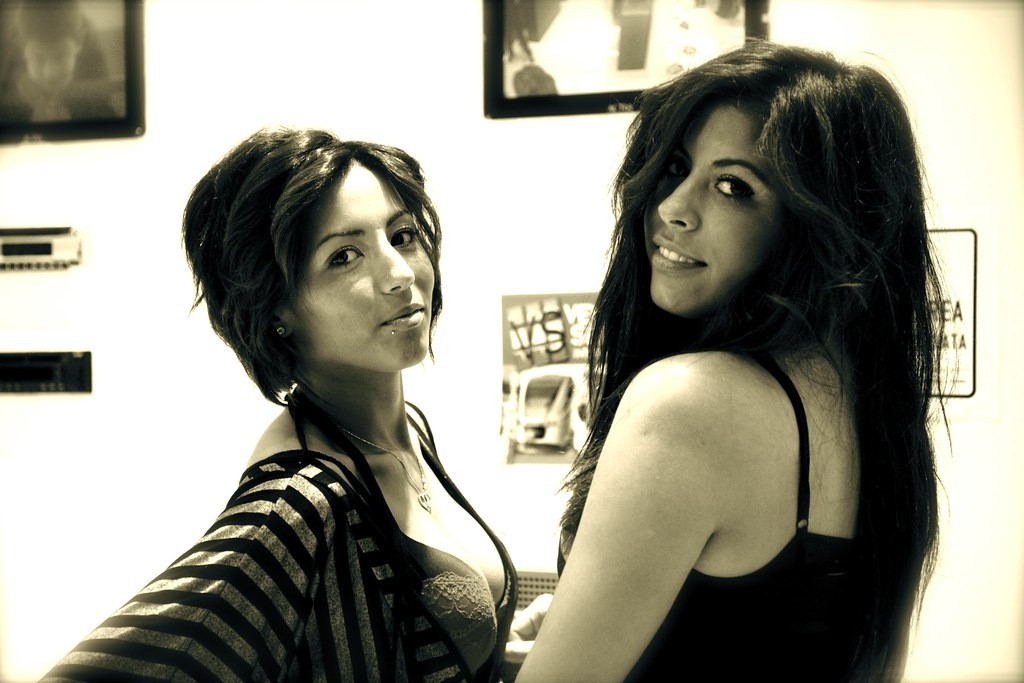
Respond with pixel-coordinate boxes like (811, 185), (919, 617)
(329, 417), (433, 514)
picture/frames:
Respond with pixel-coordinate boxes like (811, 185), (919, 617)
(481, 0), (771, 120)
(0, 0), (146, 146)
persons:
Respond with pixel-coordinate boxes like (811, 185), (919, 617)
(515, 41), (940, 683)
(0, 0), (120, 125)
(41, 126), (521, 682)
